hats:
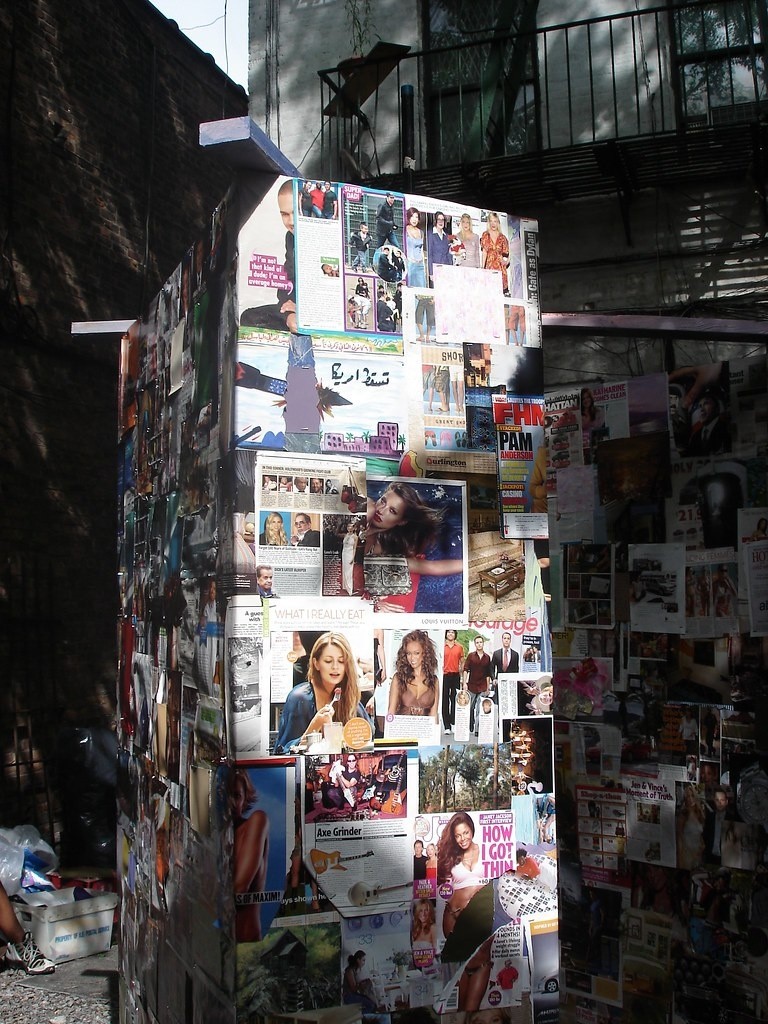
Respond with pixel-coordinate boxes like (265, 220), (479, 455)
(348, 295), (354, 301)
(387, 192), (395, 198)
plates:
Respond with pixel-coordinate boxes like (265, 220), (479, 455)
(491, 567), (505, 575)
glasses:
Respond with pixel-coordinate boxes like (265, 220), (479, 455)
(295, 521), (308, 526)
(390, 197), (394, 199)
(347, 759), (356, 763)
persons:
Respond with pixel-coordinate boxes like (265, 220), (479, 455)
(545, 358), (768, 1024)
(422, 363), (464, 413)
(117, 191), (226, 1024)
(236, 427), (556, 1024)
(240, 178), (526, 346)
(0, 880), (56, 974)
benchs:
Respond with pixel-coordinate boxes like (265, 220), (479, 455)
(609, 124), (767, 247)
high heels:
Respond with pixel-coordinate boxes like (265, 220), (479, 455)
(455, 407), (459, 414)
(460, 407), (464, 414)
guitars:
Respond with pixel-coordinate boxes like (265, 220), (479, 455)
(329, 759), (357, 808)
(377, 753), (387, 784)
(381, 767), (405, 815)
(368, 775), (386, 811)
(388, 751), (407, 783)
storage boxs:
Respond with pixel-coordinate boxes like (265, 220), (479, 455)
(8, 875), (121, 965)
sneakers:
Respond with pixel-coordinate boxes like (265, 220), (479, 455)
(1, 932), (56, 976)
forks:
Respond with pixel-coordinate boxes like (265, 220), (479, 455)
(329, 688), (341, 707)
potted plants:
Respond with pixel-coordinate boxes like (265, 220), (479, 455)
(338, 0), (381, 83)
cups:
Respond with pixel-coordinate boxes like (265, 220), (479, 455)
(305, 729), (322, 750)
(323, 721), (343, 754)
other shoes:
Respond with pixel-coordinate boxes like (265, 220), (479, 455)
(426, 337), (430, 344)
(416, 337), (425, 342)
(351, 267), (358, 273)
(362, 270), (368, 273)
(428, 408), (433, 412)
(435, 408), (450, 411)
